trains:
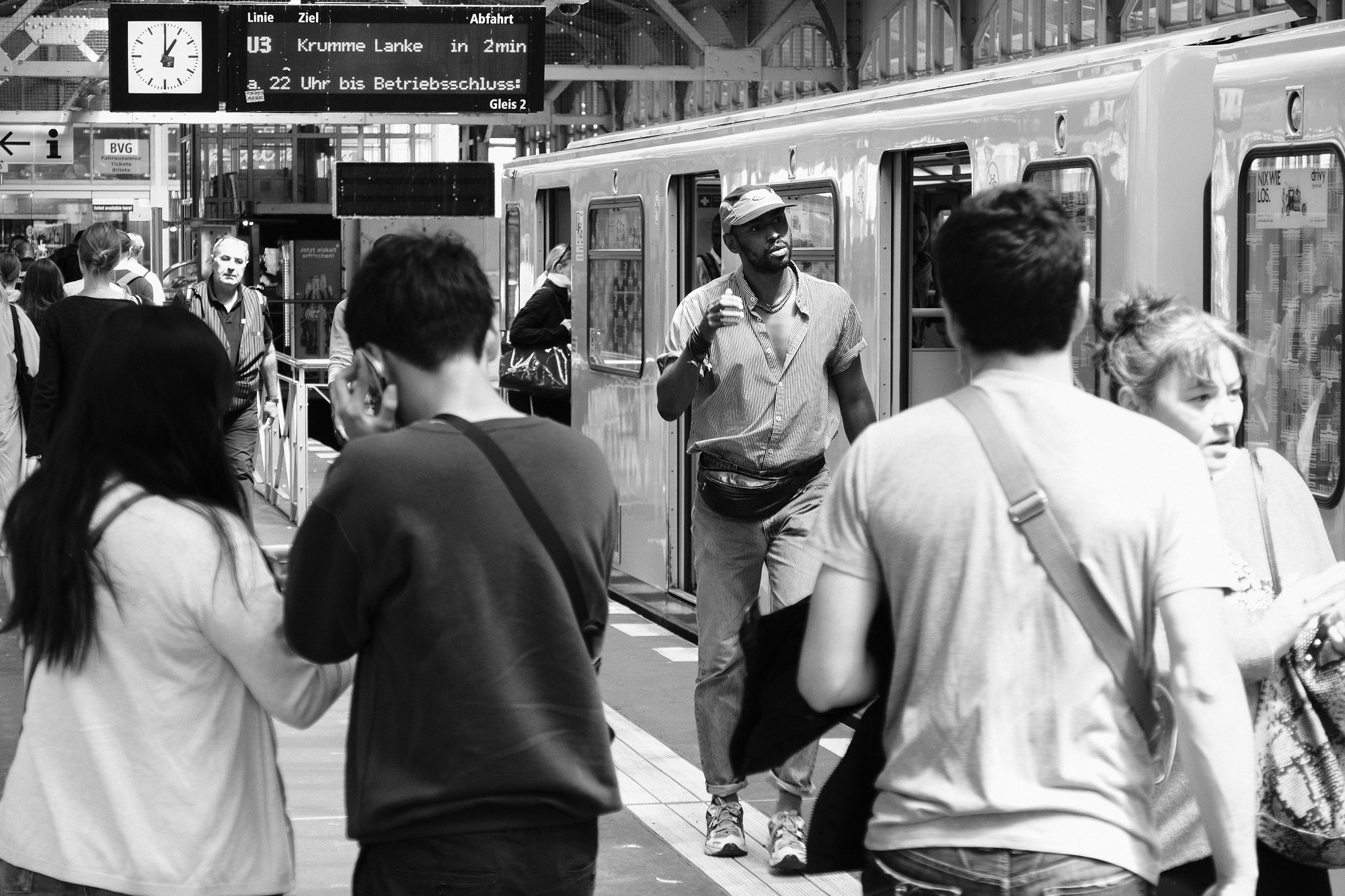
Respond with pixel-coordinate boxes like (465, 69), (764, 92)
(497, 10), (1345, 610)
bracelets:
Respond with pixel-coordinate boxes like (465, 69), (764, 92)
(686, 324), (712, 368)
(266, 396), (278, 404)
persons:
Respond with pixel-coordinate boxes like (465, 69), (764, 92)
(0, 219), (167, 634)
(1083, 285), (1345, 894)
(796, 183), (1261, 895)
(656, 184), (877, 869)
(0, 303), (360, 896)
(167, 235), (279, 535)
(509, 242), (572, 426)
(693, 213), (722, 289)
(327, 233), (399, 448)
(284, 230), (623, 896)
(913, 203), (933, 348)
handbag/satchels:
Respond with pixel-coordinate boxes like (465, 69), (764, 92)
(16, 363), (35, 434)
(1191, 613), (1345, 870)
(696, 457), (826, 520)
(1150, 683), (1179, 806)
(498, 329), (571, 398)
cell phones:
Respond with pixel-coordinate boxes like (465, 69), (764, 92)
(359, 348), (387, 416)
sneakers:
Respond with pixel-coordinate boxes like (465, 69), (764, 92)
(705, 796), (748, 856)
(765, 808), (808, 869)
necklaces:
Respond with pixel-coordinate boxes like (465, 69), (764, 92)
(754, 267), (795, 313)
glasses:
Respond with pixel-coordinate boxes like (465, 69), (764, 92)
(554, 242), (571, 269)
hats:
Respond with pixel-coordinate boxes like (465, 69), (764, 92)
(719, 185), (798, 235)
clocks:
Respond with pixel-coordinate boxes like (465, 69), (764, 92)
(107, 3), (224, 111)
